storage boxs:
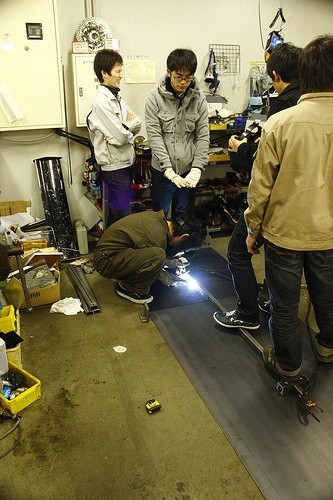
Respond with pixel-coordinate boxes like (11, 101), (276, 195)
(0, 277), (29, 309)
(20, 267), (60, 308)
(209, 152), (231, 165)
(9, 239), (59, 274)
(0, 305), (17, 333)
(0, 360), (42, 414)
(0, 200), (31, 216)
(6, 308), (22, 369)
(209, 123), (227, 133)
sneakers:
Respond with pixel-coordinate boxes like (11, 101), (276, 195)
(310, 327), (333, 357)
(257, 296), (273, 315)
(116, 280), (154, 304)
(262, 344), (303, 377)
(212, 307), (261, 329)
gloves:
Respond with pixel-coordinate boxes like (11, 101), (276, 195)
(163, 167), (190, 189)
(183, 167), (202, 188)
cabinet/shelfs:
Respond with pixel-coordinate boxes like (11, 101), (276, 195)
(101, 154), (255, 233)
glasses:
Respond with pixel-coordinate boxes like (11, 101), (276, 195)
(172, 75), (193, 81)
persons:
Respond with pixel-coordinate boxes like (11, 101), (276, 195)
(144, 49), (210, 257)
(87, 49), (143, 228)
(94, 210), (203, 304)
(212, 43), (304, 329)
(243, 34), (333, 385)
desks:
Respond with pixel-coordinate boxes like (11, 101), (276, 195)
(8, 245), (34, 312)
